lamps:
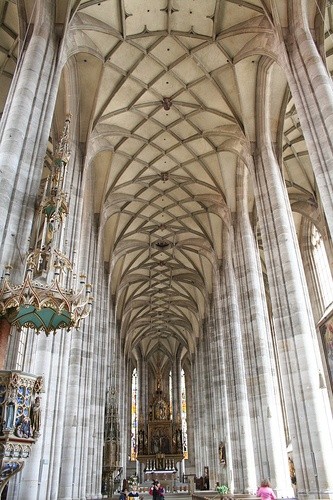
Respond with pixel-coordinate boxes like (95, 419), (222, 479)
(162, 97), (173, 110)
(0, 369), (46, 500)
(0, 113), (95, 338)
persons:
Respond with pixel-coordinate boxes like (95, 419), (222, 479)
(119, 488), (129, 500)
(128, 487), (139, 500)
(149, 480), (165, 500)
(257, 480), (276, 500)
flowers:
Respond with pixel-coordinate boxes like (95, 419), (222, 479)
(217, 485), (230, 496)
